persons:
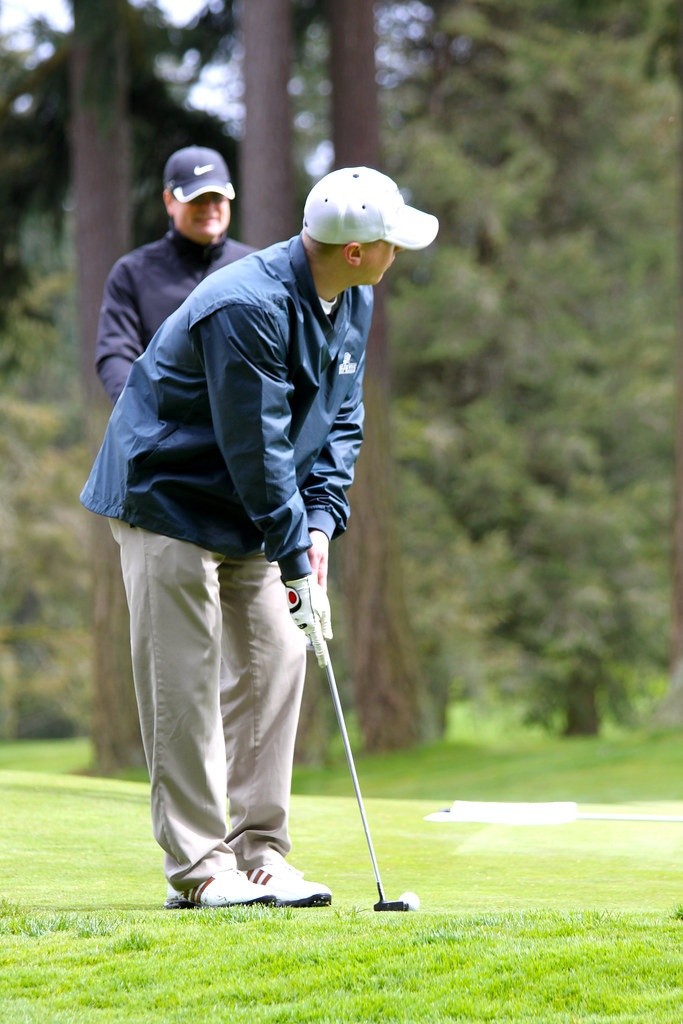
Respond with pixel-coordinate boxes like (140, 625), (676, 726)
(95, 146), (266, 406)
(78, 165), (440, 907)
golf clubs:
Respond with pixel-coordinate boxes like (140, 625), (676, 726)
(320, 645), (411, 913)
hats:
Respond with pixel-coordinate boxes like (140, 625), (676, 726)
(161, 145), (235, 204)
(302, 166), (440, 252)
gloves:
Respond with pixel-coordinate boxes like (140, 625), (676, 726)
(284, 573), (333, 668)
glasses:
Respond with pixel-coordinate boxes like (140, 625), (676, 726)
(191, 192), (227, 204)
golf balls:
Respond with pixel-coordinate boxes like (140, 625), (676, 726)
(399, 890), (419, 911)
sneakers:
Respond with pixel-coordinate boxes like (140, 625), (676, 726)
(165, 863), (332, 910)
